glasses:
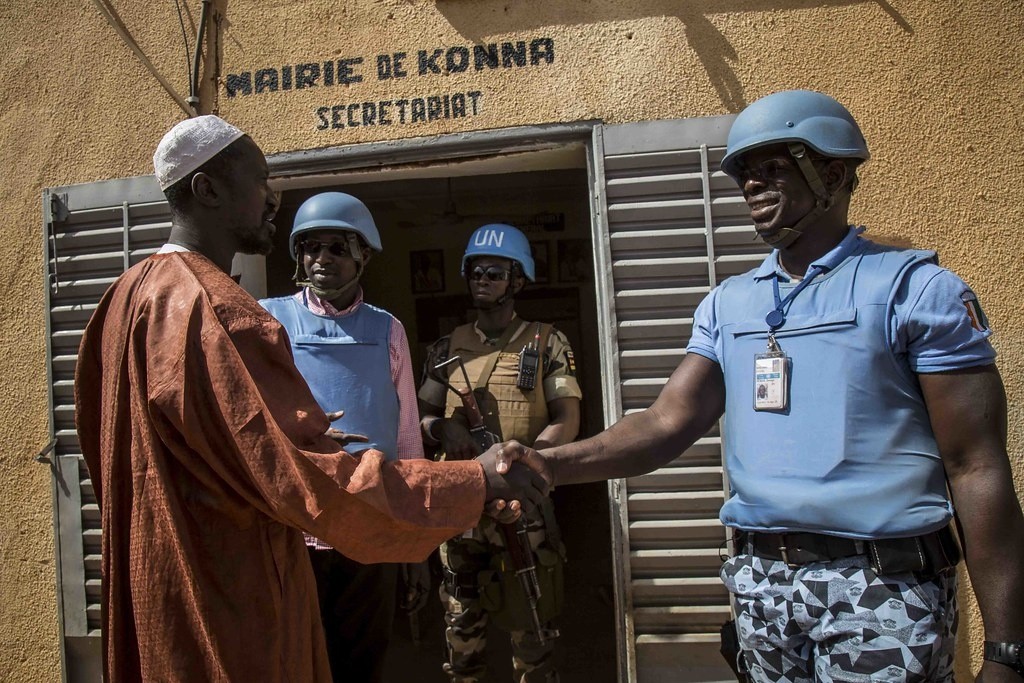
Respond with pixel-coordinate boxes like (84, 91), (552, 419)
(736, 156), (825, 188)
(470, 265), (514, 281)
(299, 240), (362, 257)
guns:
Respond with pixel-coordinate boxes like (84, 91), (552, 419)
(427, 354), (563, 650)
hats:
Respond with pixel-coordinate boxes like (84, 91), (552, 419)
(153, 115), (245, 192)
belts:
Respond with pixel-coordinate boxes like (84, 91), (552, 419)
(734, 531), (866, 569)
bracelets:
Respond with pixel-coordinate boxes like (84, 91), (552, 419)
(981, 637), (1024, 672)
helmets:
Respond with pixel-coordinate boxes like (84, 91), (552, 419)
(721, 89), (871, 177)
(460, 224), (536, 284)
(289, 192), (383, 260)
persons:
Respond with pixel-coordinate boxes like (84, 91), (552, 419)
(254, 189), (423, 683)
(481, 89), (1024, 683)
(71, 114), (549, 683)
(418, 222), (582, 683)
(757, 385), (766, 398)
